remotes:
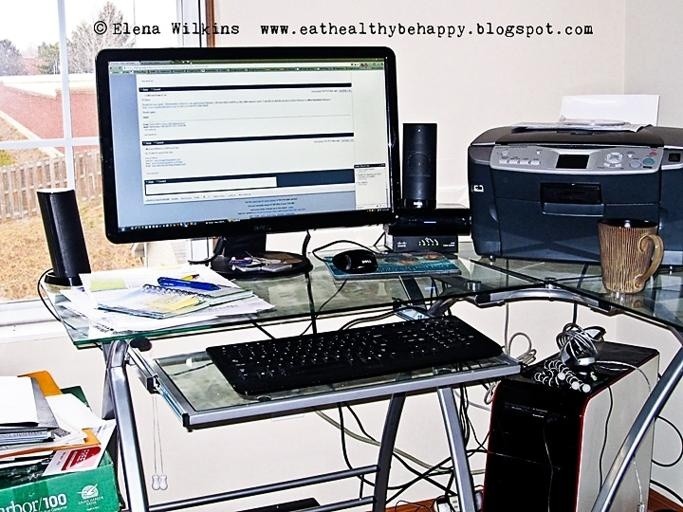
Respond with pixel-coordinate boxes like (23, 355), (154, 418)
(332, 249), (378, 274)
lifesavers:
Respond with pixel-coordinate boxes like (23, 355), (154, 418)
(479, 338), (659, 512)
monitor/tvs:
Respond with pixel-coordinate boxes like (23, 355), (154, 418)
(0, 368), (115, 486)
(97, 279), (254, 321)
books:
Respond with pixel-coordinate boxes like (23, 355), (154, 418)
(0, 385), (121, 511)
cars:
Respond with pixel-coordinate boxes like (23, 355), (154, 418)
(597, 216), (664, 294)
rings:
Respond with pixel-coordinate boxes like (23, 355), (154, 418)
(157, 274), (221, 291)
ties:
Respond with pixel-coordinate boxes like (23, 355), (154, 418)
(95, 45), (401, 278)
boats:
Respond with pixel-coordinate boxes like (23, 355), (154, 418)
(402, 123), (438, 214)
(36, 185), (91, 287)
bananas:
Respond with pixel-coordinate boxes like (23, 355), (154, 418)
(205, 315), (502, 399)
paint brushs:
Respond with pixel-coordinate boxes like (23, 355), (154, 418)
(434, 492), (483, 512)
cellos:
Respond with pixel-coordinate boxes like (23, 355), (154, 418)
(467, 119), (683, 281)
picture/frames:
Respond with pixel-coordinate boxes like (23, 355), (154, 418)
(38, 240), (683, 512)
